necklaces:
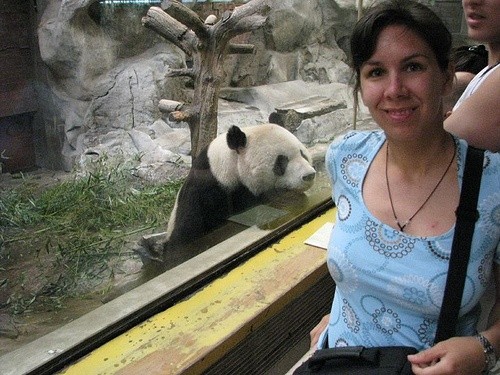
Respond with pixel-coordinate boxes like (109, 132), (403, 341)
(385, 133), (457, 232)
(470, 60), (500, 96)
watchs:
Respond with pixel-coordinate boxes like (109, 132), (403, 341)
(474, 332), (497, 375)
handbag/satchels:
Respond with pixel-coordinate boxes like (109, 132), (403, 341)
(292, 345), (420, 375)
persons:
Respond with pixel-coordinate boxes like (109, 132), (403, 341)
(309, 0), (500, 375)
